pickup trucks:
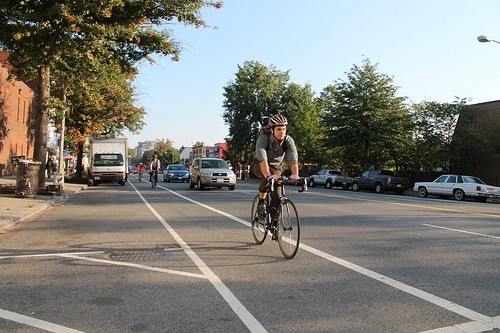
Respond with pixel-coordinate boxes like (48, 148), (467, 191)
(308, 169), (353, 191)
(351, 169), (411, 194)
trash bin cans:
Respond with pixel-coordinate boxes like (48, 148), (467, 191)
(14, 160), (42, 198)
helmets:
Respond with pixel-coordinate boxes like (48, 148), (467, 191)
(268, 114), (288, 125)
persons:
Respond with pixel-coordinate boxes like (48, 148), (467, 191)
(253, 114), (299, 240)
(149, 154), (161, 186)
(46, 156), (76, 178)
(134, 163), (146, 181)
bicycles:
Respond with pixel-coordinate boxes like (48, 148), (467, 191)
(250, 175), (307, 260)
(148, 170), (159, 188)
(139, 172), (142, 182)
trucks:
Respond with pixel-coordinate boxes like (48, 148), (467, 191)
(88, 137), (130, 186)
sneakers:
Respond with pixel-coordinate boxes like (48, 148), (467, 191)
(257, 204), (266, 218)
(272, 230), (281, 241)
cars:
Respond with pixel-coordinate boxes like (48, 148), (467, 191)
(280, 175), (305, 185)
(163, 165), (190, 182)
(189, 157), (237, 191)
(413, 175), (500, 201)
(128, 166), (137, 173)
